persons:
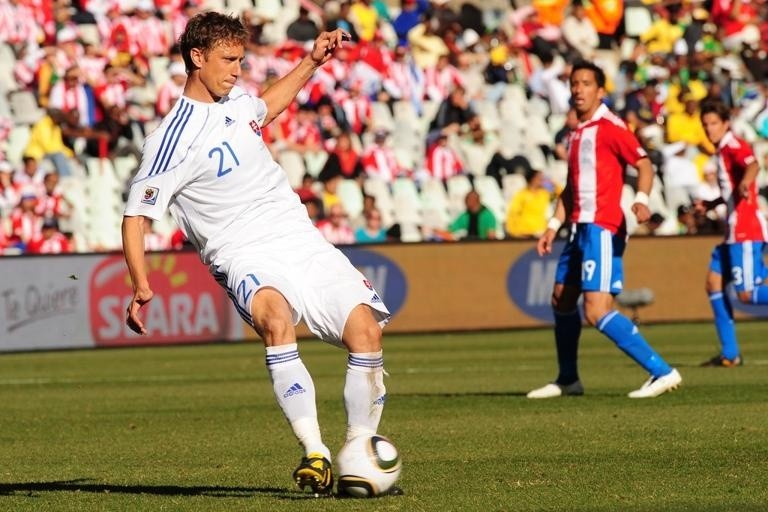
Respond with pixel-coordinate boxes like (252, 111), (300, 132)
(692, 95), (768, 368)
(526, 57), (685, 401)
(2, 0), (767, 258)
(117, 12), (393, 494)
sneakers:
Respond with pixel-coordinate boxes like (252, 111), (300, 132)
(294, 454), (333, 496)
(628, 368), (681, 398)
(698, 354), (742, 367)
(335, 481), (404, 496)
(528, 379), (583, 398)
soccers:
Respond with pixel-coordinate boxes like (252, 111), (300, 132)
(335, 433), (402, 496)
